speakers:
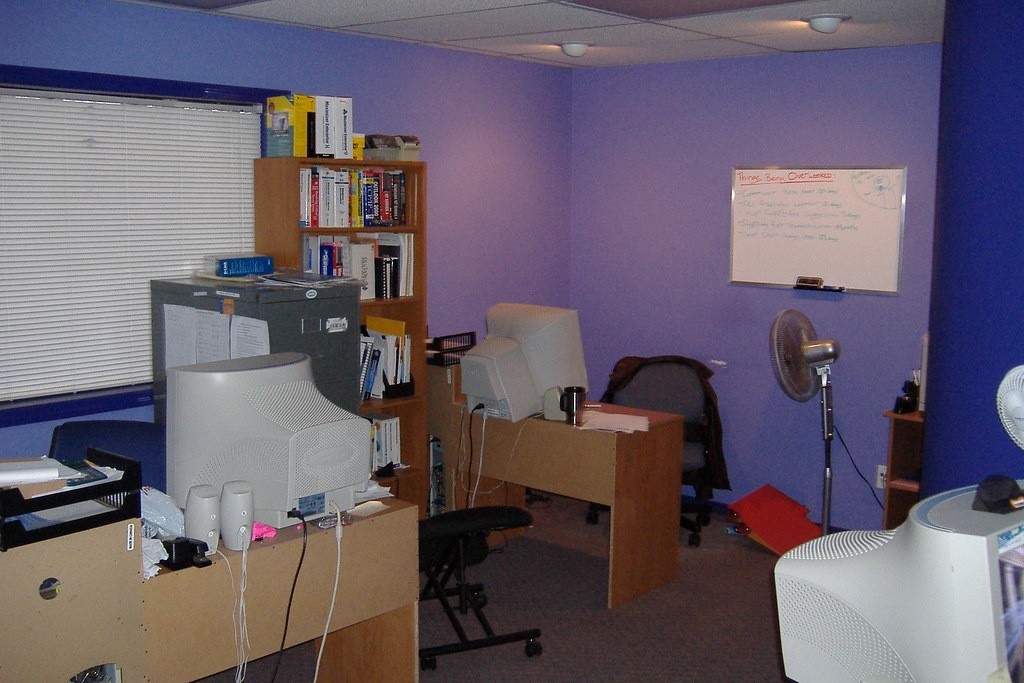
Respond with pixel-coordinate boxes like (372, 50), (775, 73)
(219, 479), (253, 551)
(184, 483), (221, 556)
(543, 386), (569, 421)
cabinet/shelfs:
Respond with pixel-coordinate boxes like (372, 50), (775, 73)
(152, 275), (362, 426)
(882, 408), (926, 531)
(252, 157), (428, 521)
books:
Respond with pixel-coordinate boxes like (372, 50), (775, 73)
(303, 232), (414, 299)
(361, 329), (411, 400)
(299, 166), (406, 227)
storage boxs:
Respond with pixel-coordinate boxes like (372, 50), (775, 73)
(204, 253), (273, 277)
(260, 94), (366, 160)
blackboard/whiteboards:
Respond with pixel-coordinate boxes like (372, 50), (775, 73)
(730, 165), (908, 296)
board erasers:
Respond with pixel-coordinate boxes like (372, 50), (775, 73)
(795, 276), (823, 287)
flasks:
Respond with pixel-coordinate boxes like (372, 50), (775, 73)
(559, 386), (586, 427)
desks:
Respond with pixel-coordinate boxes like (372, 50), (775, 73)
(425, 337), (687, 610)
(0, 498), (419, 683)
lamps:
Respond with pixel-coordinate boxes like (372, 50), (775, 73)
(801, 14), (852, 34)
(554, 41), (597, 57)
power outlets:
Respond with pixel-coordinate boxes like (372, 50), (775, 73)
(876, 465), (887, 489)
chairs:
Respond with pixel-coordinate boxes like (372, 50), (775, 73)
(419, 506), (543, 671)
(586, 355), (733, 547)
(49, 420), (166, 495)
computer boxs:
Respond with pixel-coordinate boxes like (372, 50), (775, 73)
(429, 436), (450, 519)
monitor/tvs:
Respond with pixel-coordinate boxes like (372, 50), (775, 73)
(773, 478), (1024, 683)
(166, 351), (372, 529)
(461, 302), (590, 423)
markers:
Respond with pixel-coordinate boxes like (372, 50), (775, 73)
(819, 285), (845, 292)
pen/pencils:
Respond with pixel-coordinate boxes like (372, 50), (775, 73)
(584, 403), (602, 409)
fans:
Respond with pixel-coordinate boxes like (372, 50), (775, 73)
(996, 365), (1024, 451)
(769, 307), (885, 537)
